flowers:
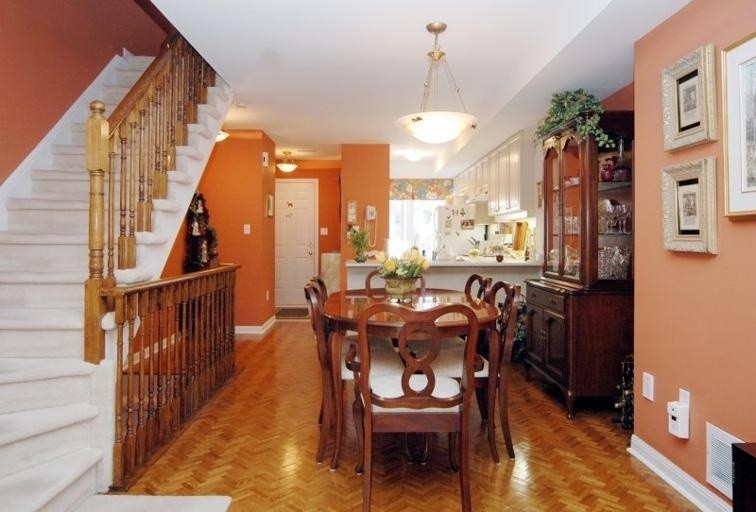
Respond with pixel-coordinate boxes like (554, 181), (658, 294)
(373, 247), (430, 278)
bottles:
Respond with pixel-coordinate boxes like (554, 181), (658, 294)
(494, 231), (501, 255)
(433, 250), (436, 261)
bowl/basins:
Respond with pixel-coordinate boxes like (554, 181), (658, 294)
(569, 177), (579, 184)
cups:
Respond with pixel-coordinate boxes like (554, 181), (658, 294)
(565, 216), (579, 235)
(496, 255), (503, 262)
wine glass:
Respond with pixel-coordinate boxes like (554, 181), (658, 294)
(599, 204), (631, 233)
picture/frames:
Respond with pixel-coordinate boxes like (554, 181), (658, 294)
(264, 191), (275, 219)
(659, 40), (716, 255)
(720, 32), (756, 221)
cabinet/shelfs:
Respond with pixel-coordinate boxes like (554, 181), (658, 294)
(451, 135), (536, 215)
(544, 110), (634, 287)
(518, 278), (635, 419)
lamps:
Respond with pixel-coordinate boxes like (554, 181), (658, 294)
(397, 19), (478, 144)
(277, 151), (298, 173)
